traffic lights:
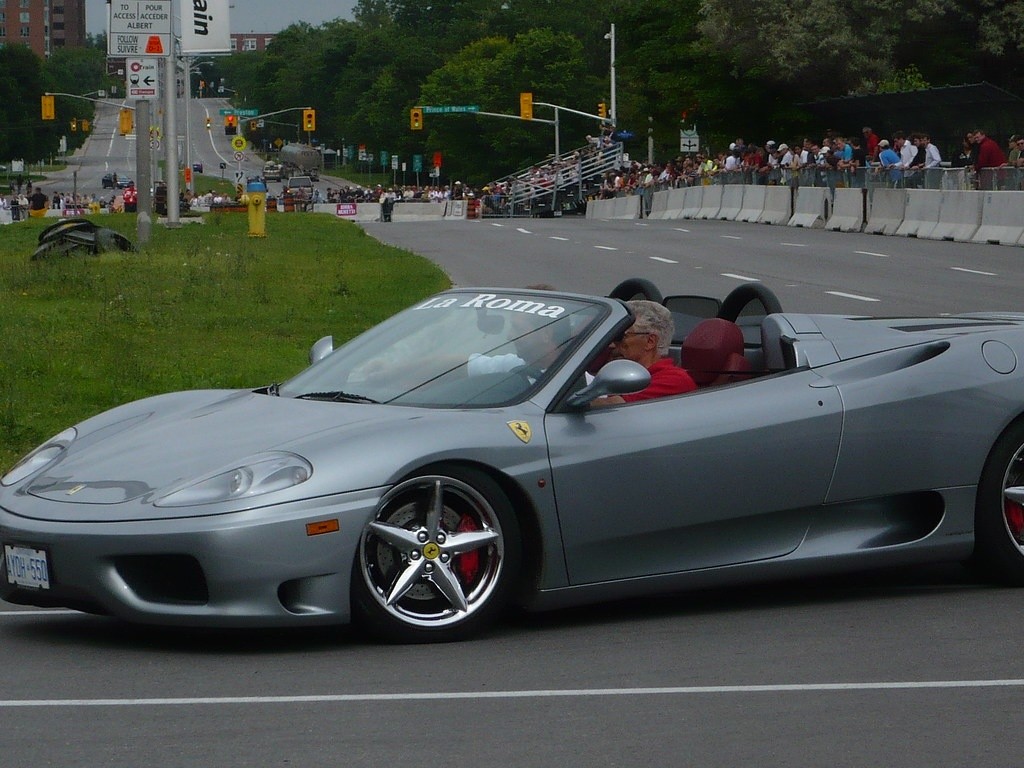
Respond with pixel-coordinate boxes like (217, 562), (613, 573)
(409, 108), (422, 131)
(251, 121), (257, 131)
(224, 116), (239, 136)
(205, 117), (212, 129)
(70, 118), (76, 131)
(598, 103), (607, 118)
(303, 110), (316, 131)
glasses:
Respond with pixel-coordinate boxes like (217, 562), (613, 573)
(1016, 143), (1023, 146)
(613, 331), (650, 343)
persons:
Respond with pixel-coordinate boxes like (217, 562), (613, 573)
(29, 187), (49, 217)
(156, 179), (167, 213)
(10, 174), (32, 195)
(51, 191), (116, 210)
(313, 184), (475, 222)
(598, 126), (1024, 199)
(591, 300), (697, 407)
(186, 189), (230, 206)
(364, 283), (608, 398)
(123, 180), (138, 213)
(0, 190), (32, 220)
(474, 124), (618, 213)
(112, 172), (117, 190)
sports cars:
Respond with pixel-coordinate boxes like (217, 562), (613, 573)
(0, 286), (1024, 644)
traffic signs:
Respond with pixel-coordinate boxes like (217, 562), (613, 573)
(218, 108), (259, 117)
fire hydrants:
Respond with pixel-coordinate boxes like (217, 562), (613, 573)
(236, 181), (270, 238)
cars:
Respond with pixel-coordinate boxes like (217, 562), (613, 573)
(286, 176), (314, 203)
(102, 173), (134, 190)
(262, 164), (282, 183)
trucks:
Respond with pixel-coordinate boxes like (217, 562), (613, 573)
(278, 142), (321, 182)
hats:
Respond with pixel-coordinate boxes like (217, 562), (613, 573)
(729, 143), (736, 150)
(878, 140), (889, 146)
(765, 141), (775, 147)
(777, 143), (788, 151)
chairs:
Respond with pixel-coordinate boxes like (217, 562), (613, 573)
(680, 317), (748, 388)
